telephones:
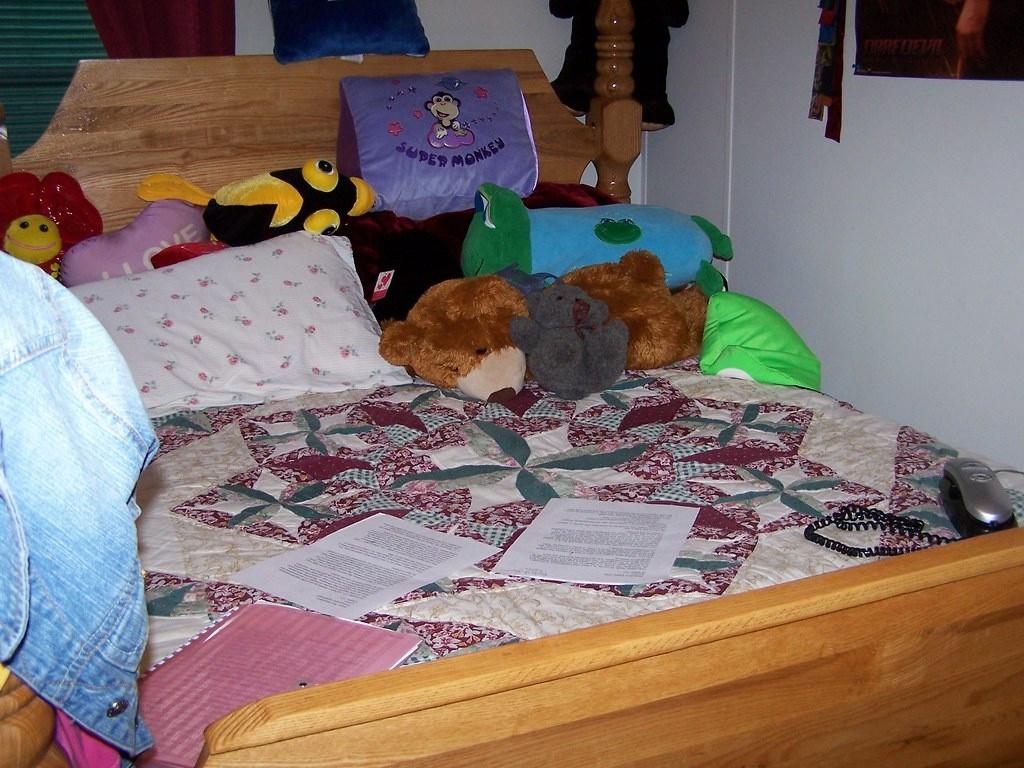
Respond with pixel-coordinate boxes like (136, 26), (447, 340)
(939, 457), (1017, 540)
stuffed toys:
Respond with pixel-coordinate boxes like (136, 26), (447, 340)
(378, 249), (708, 401)
(461, 182), (734, 297)
(0, 154), (471, 339)
(510, 284), (629, 401)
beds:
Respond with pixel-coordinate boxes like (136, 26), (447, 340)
(0, 48), (1024, 768)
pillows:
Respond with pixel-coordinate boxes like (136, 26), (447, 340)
(268, 0), (430, 64)
(698, 291), (821, 391)
(342, 68), (539, 218)
(67, 231), (415, 416)
